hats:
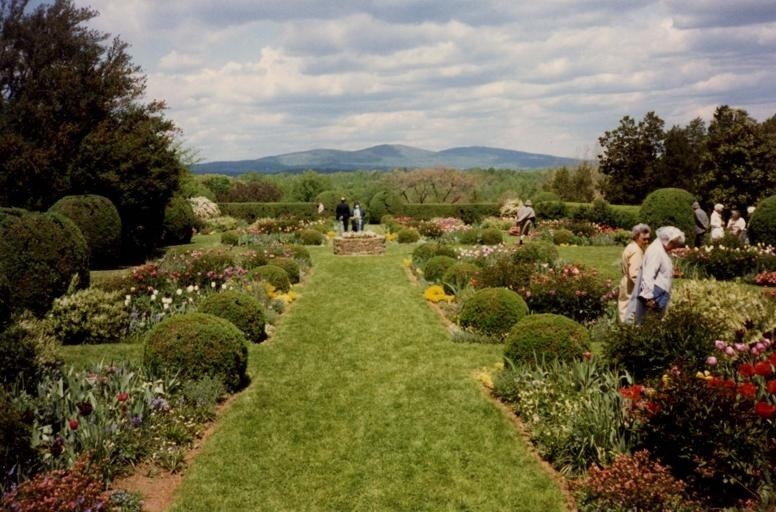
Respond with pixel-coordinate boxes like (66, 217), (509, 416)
(691, 201), (701, 208)
(524, 199), (534, 206)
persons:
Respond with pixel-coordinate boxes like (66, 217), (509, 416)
(622, 225), (685, 325)
(334, 193), (354, 233)
(692, 201), (758, 246)
(352, 201), (366, 233)
(515, 199), (537, 244)
(616, 223), (650, 320)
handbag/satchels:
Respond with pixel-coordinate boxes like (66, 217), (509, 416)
(634, 283), (671, 312)
